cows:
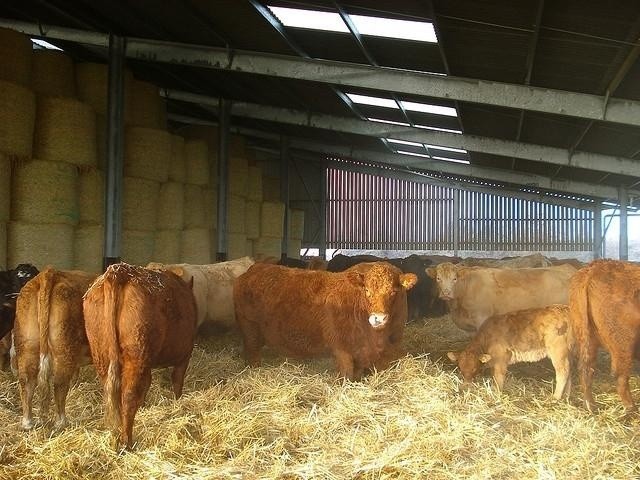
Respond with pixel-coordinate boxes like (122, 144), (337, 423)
(448, 305), (578, 406)
(275, 245), (580, 317)
(230, 262), (419, 385)
(568, 256), (640, 415)
(423, 262), (582, 337)
(80, 262), (200, 454)
(142, 251), (277, 333)
(1, 261), (92, 431)
